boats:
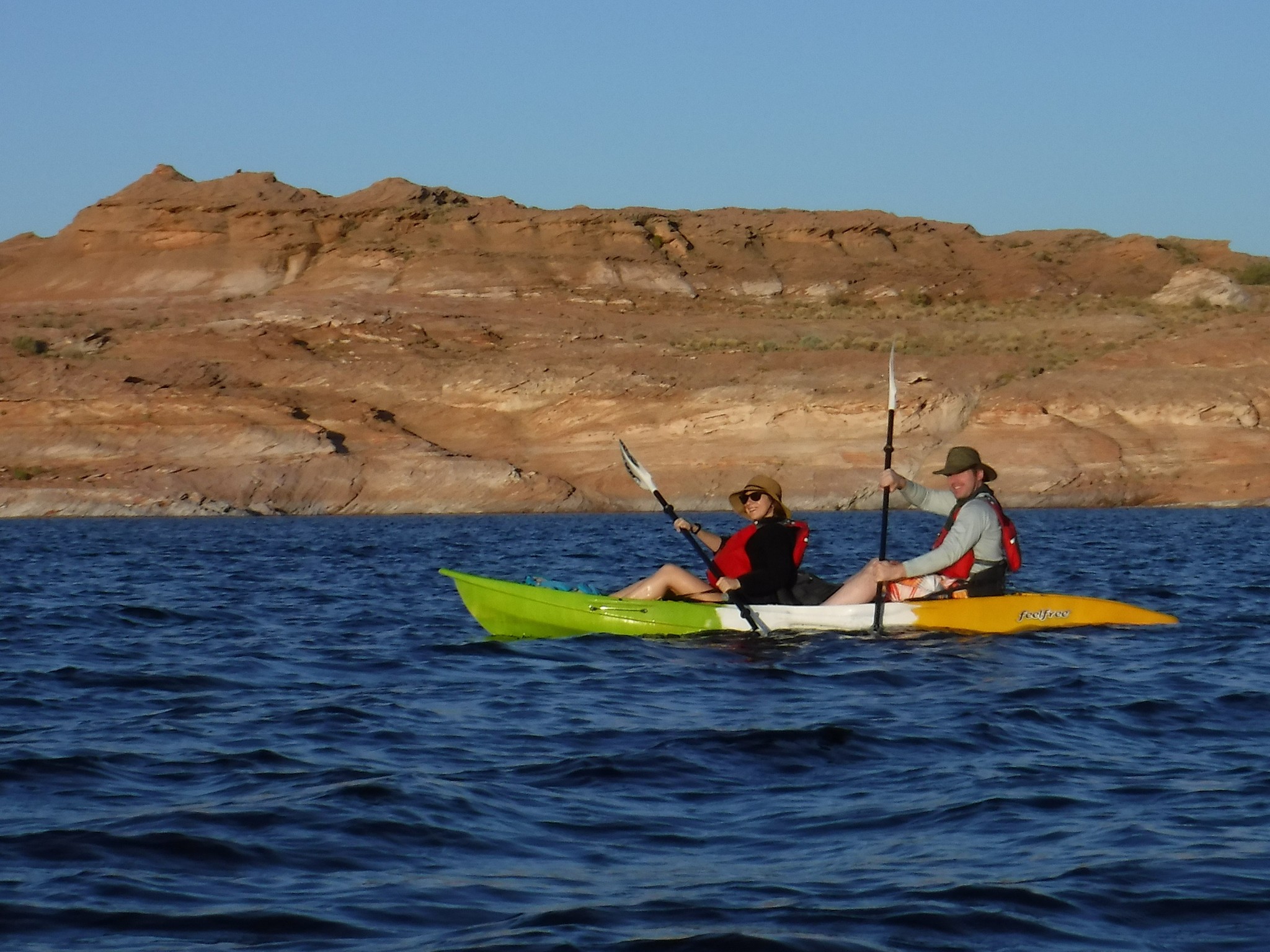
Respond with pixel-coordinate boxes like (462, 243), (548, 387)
(437, 566), (1182, 641)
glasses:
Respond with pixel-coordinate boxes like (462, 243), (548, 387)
(738, 488), (770, 505)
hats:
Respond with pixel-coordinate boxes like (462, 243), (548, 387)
(728, 475), (792, 520)
(932, 446), (998, 482)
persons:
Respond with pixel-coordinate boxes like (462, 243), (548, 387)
(608, 475), (809, 605)
(818, 446), (1022, 606)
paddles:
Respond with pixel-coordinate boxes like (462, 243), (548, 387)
(618, 438), (759, 631)
(872, 340), (898, 631)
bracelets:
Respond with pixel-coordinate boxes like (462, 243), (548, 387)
(690, 523), (701, 535)
